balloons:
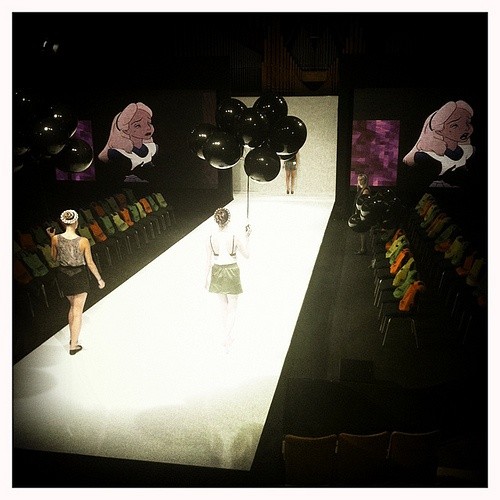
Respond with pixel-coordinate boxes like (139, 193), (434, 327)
(188, 93), (308, 183)
(347, 183), (404, 233)
(13, 89), (95, 179)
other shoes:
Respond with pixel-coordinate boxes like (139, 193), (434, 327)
(68, 340), (83, 355)
(287, 191), (289, 194)
(291, 191), (294, 194)
(352, 248), (366, 255)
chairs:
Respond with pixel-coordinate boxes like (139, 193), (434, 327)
(370, 190), (487, 356)
(278, 425), (439, 476)
(13, 190), (177, 319)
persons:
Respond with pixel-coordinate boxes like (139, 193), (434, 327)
(351, 173), (374, 256)
(43, 209), (107, 355)
(280, 147), (299, 195)
(204, 206), (251, 307)
